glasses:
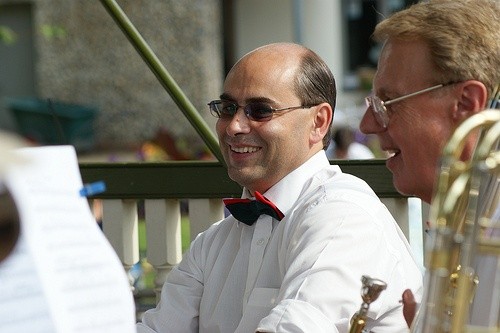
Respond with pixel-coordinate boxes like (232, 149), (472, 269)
(364, 81), (454, 130)
(207, 99), (306, 121)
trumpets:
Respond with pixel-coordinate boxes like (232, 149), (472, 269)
(347, 109), (500, 333)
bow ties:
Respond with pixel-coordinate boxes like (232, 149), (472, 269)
(222, 191), (284, 226)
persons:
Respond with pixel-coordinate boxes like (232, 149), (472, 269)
(134, 42), (424, 333)
(325, 125), (376, 159)
(360, 0), (500, 333)
